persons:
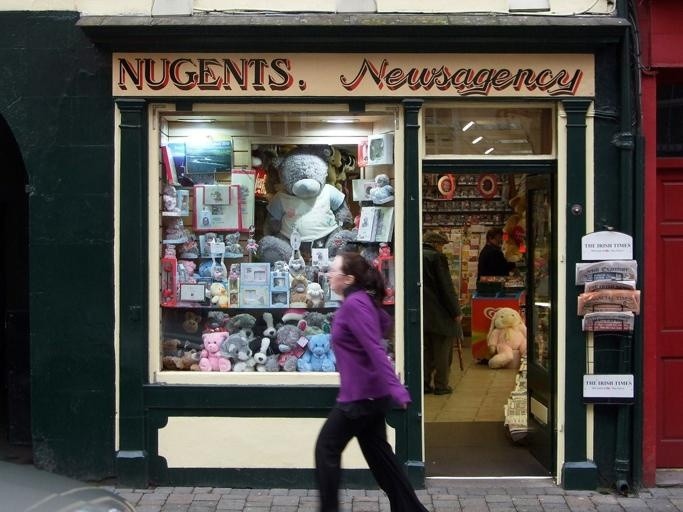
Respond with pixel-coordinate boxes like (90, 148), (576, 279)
(477, 227), (512, 282)
(422, 230), (464, 396)
(314, 250), (427, 512)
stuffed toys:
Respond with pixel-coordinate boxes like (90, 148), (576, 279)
(486, 307), (527, 370)
(162, 144), (395, 372)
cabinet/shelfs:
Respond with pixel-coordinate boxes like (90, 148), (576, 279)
(346, 161), (391, 248)
(423, 169), (512, 300)
(160, 210), (187, 245)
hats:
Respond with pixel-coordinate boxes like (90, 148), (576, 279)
(423, 231), (449, 244)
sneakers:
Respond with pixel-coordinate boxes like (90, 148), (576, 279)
(423, 384), (434, 394)
(434, 386), (452, 395)
